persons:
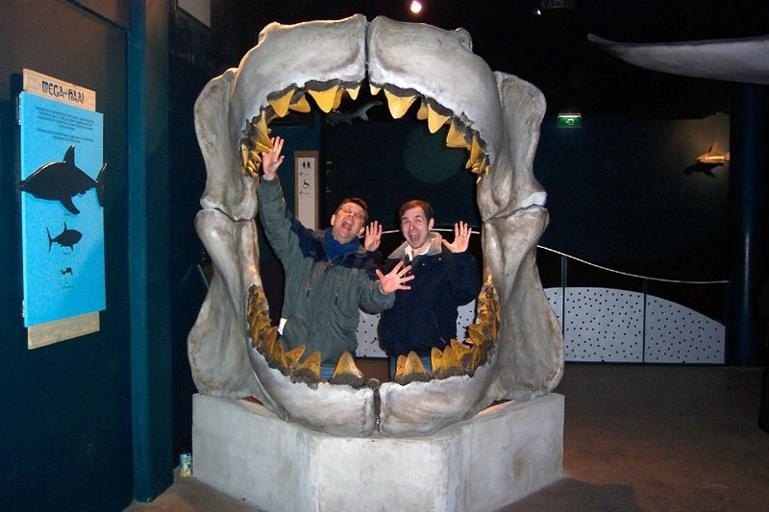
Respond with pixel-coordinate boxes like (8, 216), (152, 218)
(363, 199), (484, 382)
(256, 135), (416, 383)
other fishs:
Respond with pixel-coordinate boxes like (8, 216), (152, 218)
(695, 144), (730, 164)
(46, 222), (83, 253)
(18, 144), (108, 214)
